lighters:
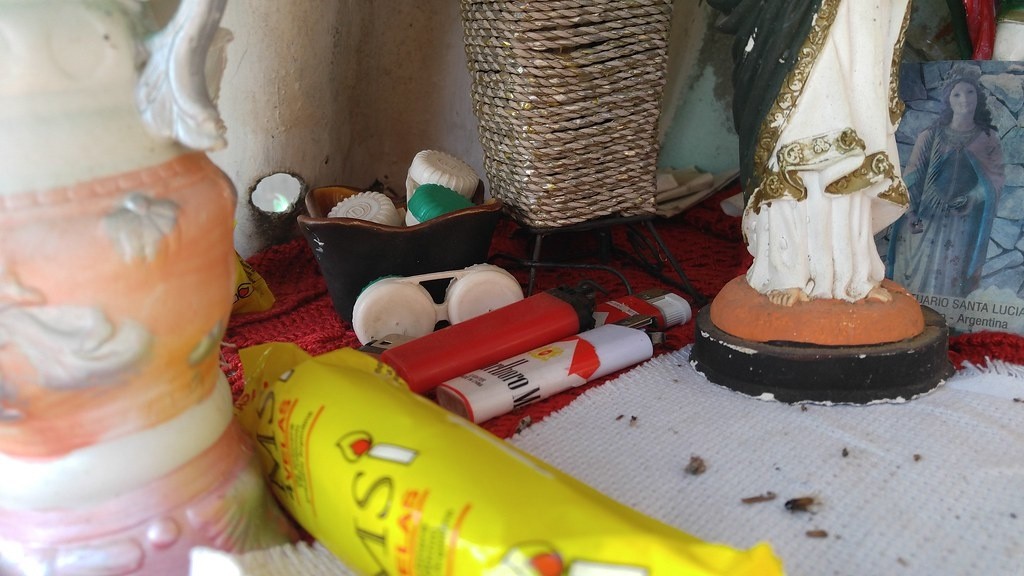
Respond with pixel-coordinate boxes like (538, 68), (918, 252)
(358, 280), (692, 424)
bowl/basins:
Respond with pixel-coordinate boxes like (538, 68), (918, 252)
(298, 179), (502, 326)
